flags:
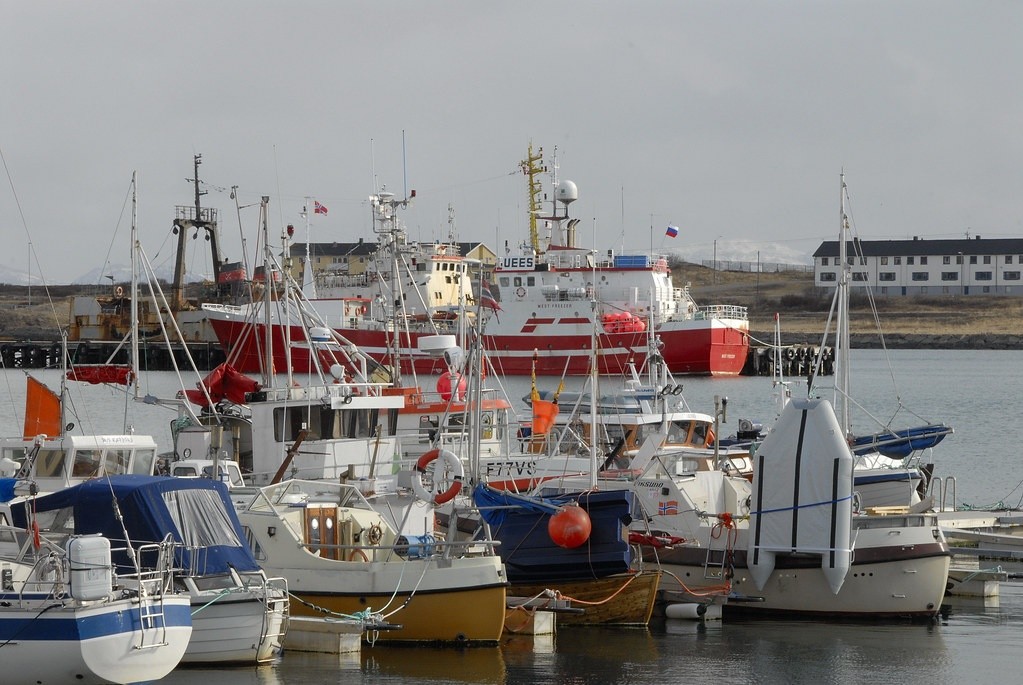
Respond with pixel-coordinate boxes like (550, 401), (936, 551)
(480, 277), (501, 310)
(665, 224), (679, 239)
(314, 200), (328, 216)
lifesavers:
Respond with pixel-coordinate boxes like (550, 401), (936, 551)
(766, 348), (779, 362)
(78, 343), (89, 356)
(117, 287), (123, 296)
(516, 287), (526, 298)
(785, 348), (796, 360)
(356, 308), (361, 316)
(26, 345), (41, 359)
(819, 348), (828, 360)
(831, 347), (836, 361)
(148, 346), (160, 359)
(334, 376), (359, 396)
(598, 469), (644, 478)
(0, 345), (11, 358)
(411, 449), (464, 504)
(797, 348), (806, 361)
(688, 305), (693, 313)
(50, 343), (62, 357)
(807, 346), (815, 361)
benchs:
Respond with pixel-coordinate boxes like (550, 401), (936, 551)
(864, 506), (910, 516)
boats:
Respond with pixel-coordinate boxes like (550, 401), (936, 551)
(473, 135), (752, 379)
(0, 158), (953, 685)
(201, 128), (486, 377)
(68, 152), (251, 344)
